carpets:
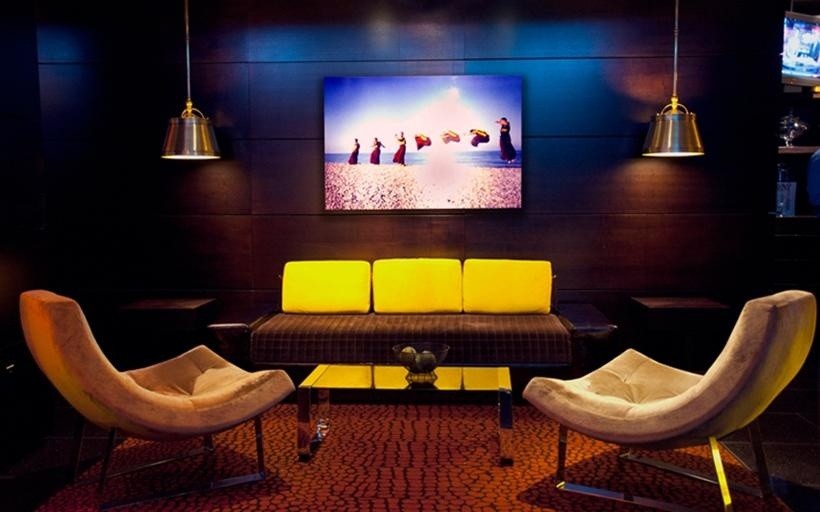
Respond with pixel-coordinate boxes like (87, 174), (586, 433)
(36, 404), (791, 512)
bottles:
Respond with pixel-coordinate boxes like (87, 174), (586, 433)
(778, 162), (789, 217)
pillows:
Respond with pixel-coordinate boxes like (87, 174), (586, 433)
(463, 258), (552, 313)
(371, 258), (463, 315)
(281, 259), (371, 314)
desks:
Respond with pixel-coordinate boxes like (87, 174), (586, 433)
(630, 296), (729, 373)
(115, 298), (219, 368)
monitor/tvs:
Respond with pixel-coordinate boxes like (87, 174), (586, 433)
(781, 11), (820, 87)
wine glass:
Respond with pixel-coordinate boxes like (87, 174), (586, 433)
(391, 342), (451, 383)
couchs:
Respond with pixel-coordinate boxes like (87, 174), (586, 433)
(207, 272), (618, 403)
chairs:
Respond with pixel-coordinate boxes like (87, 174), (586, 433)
(521, 288), (816, 512)
(18, 289), (296, 512)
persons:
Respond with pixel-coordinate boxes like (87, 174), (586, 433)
(496, 117), (516, 164)
(393, 132), (406, 165)
(370, 138), (385, 164)
(441, 129), (460, 144)
(347, 139), (360, 164)
(470, 129), (489, 146)
(415, 132), (431, 150)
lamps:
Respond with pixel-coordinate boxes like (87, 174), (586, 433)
(642, 0), (705, 159)
(160, 0), (223, 159)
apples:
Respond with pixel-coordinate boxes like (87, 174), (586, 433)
(415, 351), (436, 371)
(399, 346), (417, 363)
